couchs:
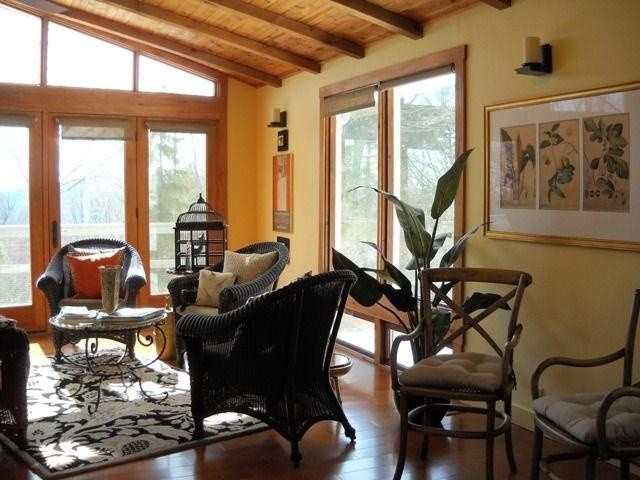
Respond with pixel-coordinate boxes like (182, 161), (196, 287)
(1, 313), (30, 450)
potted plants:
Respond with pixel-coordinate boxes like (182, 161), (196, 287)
(332, 147), (511, 426)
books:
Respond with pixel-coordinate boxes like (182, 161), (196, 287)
(55, 305), (165, 326)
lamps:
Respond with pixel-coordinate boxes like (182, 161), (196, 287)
(515, 37), (552, 74)
(267, 108), (287, 127)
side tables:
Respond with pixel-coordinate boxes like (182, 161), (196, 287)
(329, 355), (353, 408)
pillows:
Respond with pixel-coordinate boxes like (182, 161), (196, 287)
(67, 251), (124, 300)
(194, 269), (236, 308)
(65, 242), (128, 298)
(222, 249), (277, 282)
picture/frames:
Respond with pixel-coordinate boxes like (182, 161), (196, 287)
(278, 130), (288, 150)
(273, 153), (294, 232)
(276, 237), (290, 265)
(482, 81), (640, 250)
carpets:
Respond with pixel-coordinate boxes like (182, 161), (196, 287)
(1, 347), (271, 480)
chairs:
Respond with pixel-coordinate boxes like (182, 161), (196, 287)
(166, 241), (289, 366)
(532, 284), (639, 479)
(175, 270), (357, 468)
(36, 238), (146, 362)
(390, 268), (533, 479)
